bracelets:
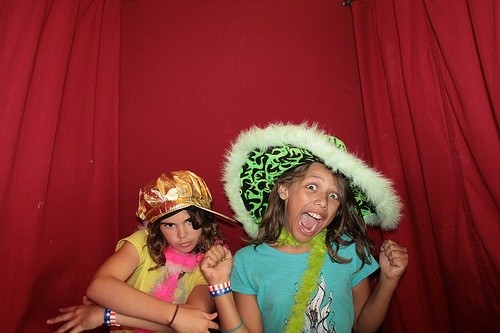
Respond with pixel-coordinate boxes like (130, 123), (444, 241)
(110, 311), (120, 326)
(209, 286), (232, 298)
(104, 308), (111, 327)
(168, 304), (179, 327)
(208, 280), (230, 292)
(220, 319), (243, 333)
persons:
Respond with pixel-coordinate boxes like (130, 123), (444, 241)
(200, 124), (408, 333)
(47, 170), (230, 333)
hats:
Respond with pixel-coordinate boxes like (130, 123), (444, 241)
(224, 120), (401, 243)
(136, 169), (236, 227)
